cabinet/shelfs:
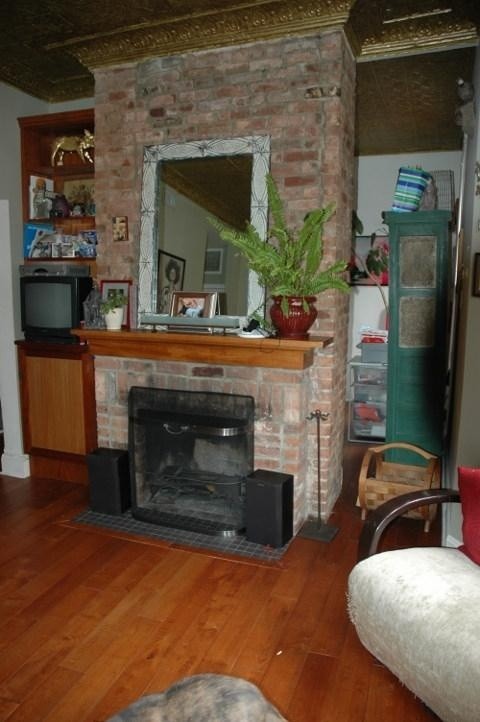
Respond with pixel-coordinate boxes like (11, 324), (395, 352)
(17, 108), (97, 260)
(382, 210), (452, 468)
(14, 339), (98, 486)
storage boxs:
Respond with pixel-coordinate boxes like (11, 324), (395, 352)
(392, 165), (431, 212)
(346, 342), (387, 445)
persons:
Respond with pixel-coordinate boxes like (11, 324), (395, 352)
(43, 240), (52, 258)
(31, 241), (45, 257)
(67, 182), (91, 216)
(160, 259), (183, 314)
(177, 297), (205, 319)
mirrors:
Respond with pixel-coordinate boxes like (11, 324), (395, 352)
(137, 134), (272, 334)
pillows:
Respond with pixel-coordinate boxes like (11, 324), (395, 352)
(457, 466), (480, 566)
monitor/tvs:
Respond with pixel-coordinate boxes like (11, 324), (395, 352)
(19, 276), (92, 342)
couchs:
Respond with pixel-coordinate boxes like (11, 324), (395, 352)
(345, 488), (480, 722)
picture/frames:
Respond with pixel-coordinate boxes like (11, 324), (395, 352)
(204, 248), (224, 274)
(168, 291), (217, 318)
(157, 249), (186, 314)
(99, 279), (133, 331)
(351, 236), (388, 286)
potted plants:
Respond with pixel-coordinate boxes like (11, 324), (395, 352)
(98, 293), (129, 330)
(203, 170), (352, 341)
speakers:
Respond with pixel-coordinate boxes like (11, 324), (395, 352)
(247, 469), (294, 548)
(88, 448), (131, 515)
